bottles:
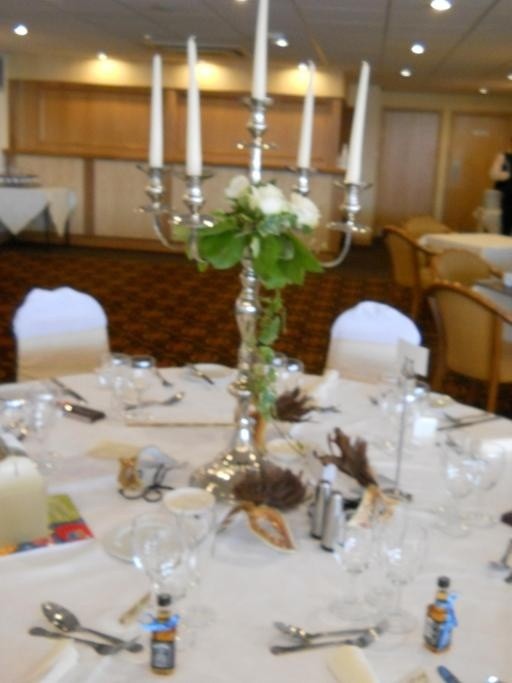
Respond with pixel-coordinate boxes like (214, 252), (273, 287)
(151, 594), (177, 676)
(422, 574), (459, 654)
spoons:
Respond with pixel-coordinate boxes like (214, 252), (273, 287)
(39, 599), (147, 654)
(271, 617), (382, 644)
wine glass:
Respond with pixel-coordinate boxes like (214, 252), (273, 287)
(330, 489), (427, 634)
(262, 350), (304, 400)
(371, 377), (428, 456)
(0, 374), (66, 471)
(99, 349), (161, 430)
(432, 433), (506, 538)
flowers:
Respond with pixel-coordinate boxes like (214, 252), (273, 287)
(193, 175), (324, 403)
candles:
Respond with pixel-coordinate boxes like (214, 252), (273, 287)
(150, 0), (373, 179)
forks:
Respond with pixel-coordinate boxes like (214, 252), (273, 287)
(270, 618), (392, 659)
(27, 626), (142, 656)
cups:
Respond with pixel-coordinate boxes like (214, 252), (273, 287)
(163, 486), (216, 542)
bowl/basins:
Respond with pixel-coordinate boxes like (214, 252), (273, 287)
(265, 436), (306, 460)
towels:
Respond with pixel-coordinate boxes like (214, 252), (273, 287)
(1, 537), (151, 683)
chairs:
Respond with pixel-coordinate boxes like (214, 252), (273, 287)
(329, 214), (512, 415)
(11, 285), (109, 380)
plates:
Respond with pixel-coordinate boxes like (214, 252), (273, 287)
(102, 519), (190, 568)
